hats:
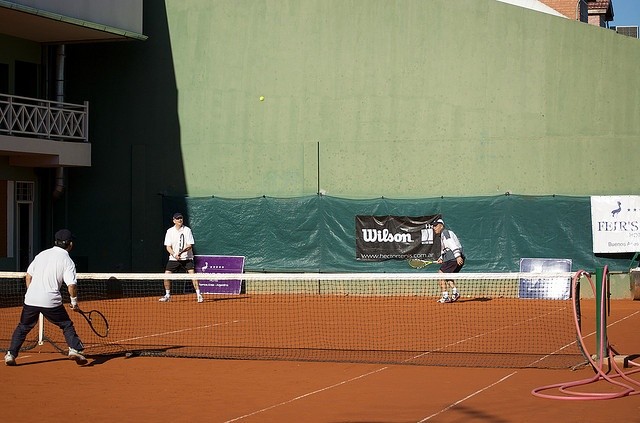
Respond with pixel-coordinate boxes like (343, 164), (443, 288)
(174, 213), (183, 218)
(430, 218), (444, 225)
(55, 229), (74, 241)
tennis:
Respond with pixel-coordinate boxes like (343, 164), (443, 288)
(259, 96), (265, 101)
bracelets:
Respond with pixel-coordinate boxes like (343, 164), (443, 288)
(70, 296), (77, 306)
(173, 252), (176, 257)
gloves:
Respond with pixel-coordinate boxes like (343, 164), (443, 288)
(70, 296), (79, 310)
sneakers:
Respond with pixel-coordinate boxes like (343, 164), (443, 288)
(198, 294), (203, 302)
(159, 294), (171, 301)
(438, 297), (449, 302)
(5, 350), (16, 365)
(68, 346), (88, 365)
(451, 293), (459, 301)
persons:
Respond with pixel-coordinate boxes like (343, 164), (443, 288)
(5, 229), (90, 365)
(430, 218), (465, 303)
(158, 212), (203, 302)
